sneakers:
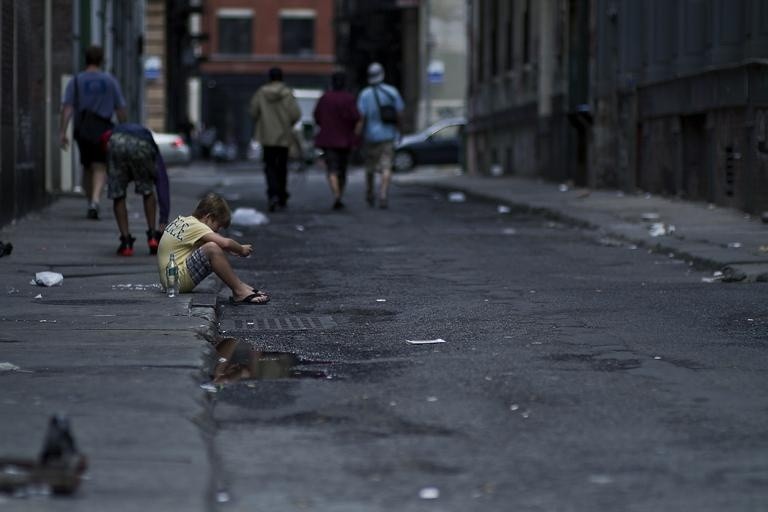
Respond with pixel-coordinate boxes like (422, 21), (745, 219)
(117, 235), (136, 256)
(146, 230), (161, 251)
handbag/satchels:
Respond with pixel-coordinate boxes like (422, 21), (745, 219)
(379, 105), (398, 125)
(73, 109), (114, 149)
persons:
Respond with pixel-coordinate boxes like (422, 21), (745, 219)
(356, 62), (406, 209)
(102, 122), (171, 257)
(156, 190), (271, 306)
(312, 71), (361, 210)
(248, 66), (302, 212)
(57, 45), (127, 220)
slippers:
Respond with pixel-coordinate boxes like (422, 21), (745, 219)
(229, 294), (270, 305)
(252, 289), (267, 295)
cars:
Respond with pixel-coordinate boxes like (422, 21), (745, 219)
(368, 118), (466, 173)
(248, 88), (335, 167)
(142, 126), (192, 167)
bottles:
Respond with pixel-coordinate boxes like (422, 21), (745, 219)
(166, 253), (180, 298)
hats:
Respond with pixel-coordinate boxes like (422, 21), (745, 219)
(367, 63), (385, 85)
